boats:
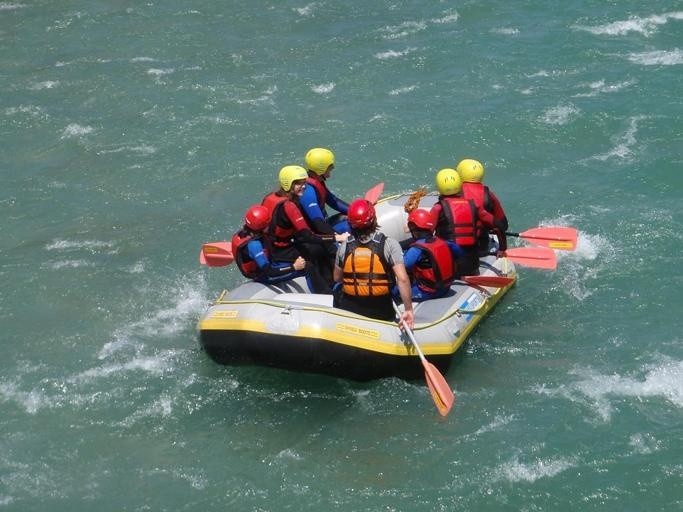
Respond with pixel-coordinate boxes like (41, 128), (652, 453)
(193, 188), (519, 385)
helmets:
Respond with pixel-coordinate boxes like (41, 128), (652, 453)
(347, 200), (376, 228)
(407, 210), (434, 231)
(305, 148), (335, 175)
(244, 204), (273, 231)
(279, 165), (308, 192)
(435, 168), (463, 195)
(457, 159), (484, 182)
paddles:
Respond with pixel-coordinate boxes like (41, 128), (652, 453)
(481, 246), (556, 270)
(392, 298), (454, 417)
(200, 242), (234, 267)
(455, 272), (513, 287)
(489, 227), (577, 251)
(364, 183), (383, 204)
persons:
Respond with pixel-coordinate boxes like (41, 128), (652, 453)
(232, 148), (351, 294)
(333, 200), (415, 334)
(391, 159), (508, 302)
